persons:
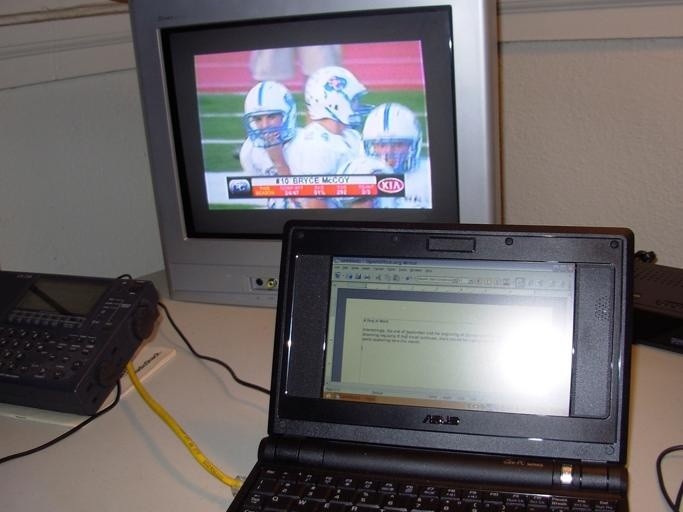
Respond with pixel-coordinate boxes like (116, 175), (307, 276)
(236, 81), (302, 209)
(299, 43), (342, 95)
(361, 103), (431, 209)
(304, 155), (397, 208)
(247, 47), (295, 88)
(262, 66), (370, 212)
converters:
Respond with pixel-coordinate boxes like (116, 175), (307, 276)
(633, 258), (683, 355)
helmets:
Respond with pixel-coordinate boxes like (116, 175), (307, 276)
(243, 81), (298, 148)
(362, 102), (424, 171)
(305, 66), (375, 127)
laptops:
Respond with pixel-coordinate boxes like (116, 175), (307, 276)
(227, 220), (635, 511)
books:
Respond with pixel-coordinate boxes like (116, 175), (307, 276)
(0, 344), (175, 427)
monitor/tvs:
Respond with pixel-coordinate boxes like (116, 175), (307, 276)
(127, 0), (500, 309)
